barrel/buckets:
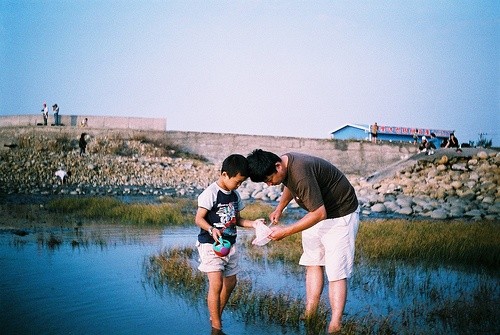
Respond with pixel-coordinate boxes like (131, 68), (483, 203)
(213, 237), (230, 256)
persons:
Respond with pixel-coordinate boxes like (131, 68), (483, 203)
(372, 122), (379, 144)
(195, 154), (265, 335)
(413, 129), (419, 146)
(80, 118), (88, 128)
(52, 104), (59, 126)
(79, 132), (87, 158)
(41, 104), (49, 126)
(417, 131), (459, 155)
(247, 148), (360, 334)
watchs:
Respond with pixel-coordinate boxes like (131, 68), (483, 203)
(209, 226), (215, 236)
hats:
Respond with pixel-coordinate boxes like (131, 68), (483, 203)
(422, 136), (426, 140)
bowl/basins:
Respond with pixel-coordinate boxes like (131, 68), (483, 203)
(251, 230), (273, 245)
